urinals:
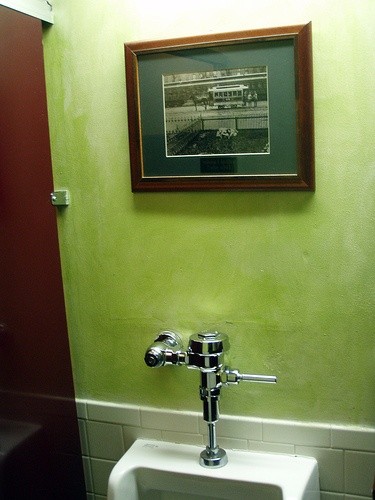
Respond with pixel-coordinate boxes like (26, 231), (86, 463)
(108, 435), (324, 500)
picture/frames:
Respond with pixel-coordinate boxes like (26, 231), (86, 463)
(123, 21), (316, 193)
